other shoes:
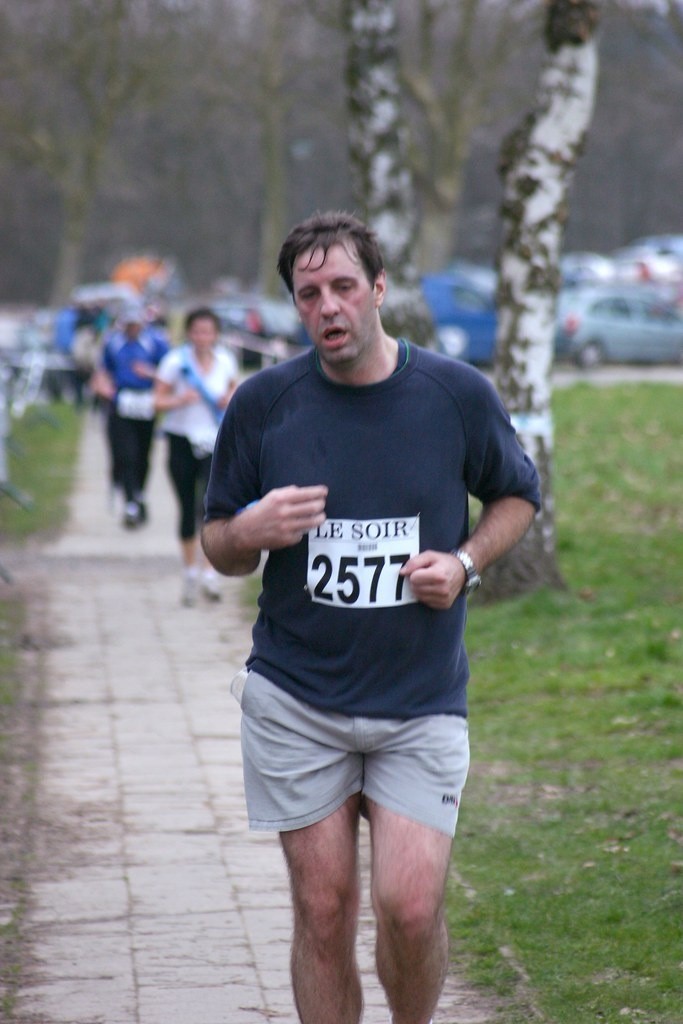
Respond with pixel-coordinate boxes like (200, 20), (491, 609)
(180, 575), (199, 608)
(123, 500), (146, 529)
(201, 573), (222, 602)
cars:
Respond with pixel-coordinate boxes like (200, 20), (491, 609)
(553, 284), (683, 372)
(419, 234), (683, 368)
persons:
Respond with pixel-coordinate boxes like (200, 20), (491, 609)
(53, 289), (263, 607)
(198, 211), (541, 1024)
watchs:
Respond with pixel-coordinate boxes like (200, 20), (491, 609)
(451, 548), (482, 596)
